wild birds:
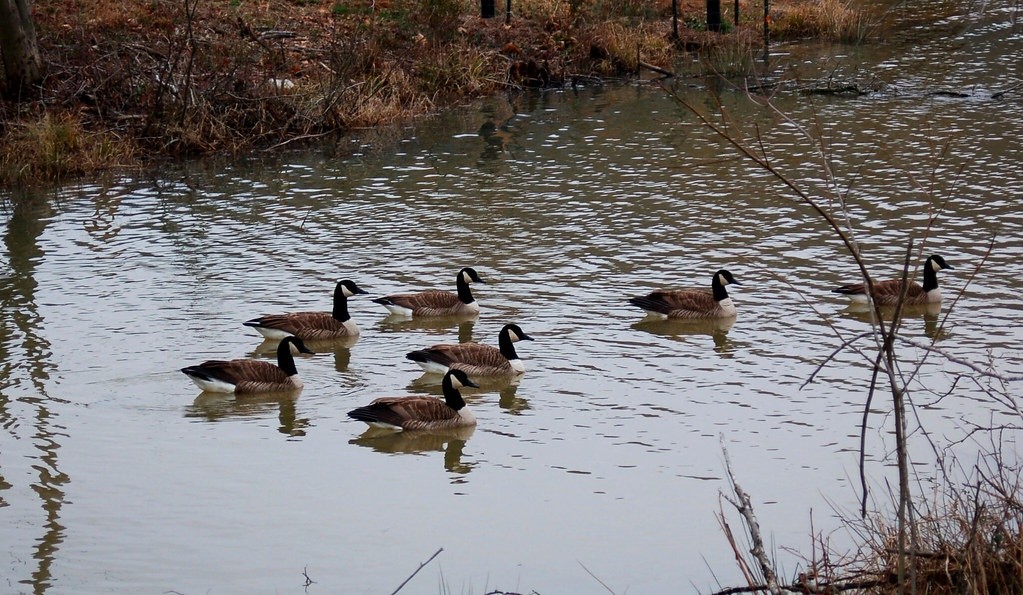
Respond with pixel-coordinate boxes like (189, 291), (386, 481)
(180, 267), (535, 431)
(831, 255), (955, 304)
(618, 270), (743, 318)
